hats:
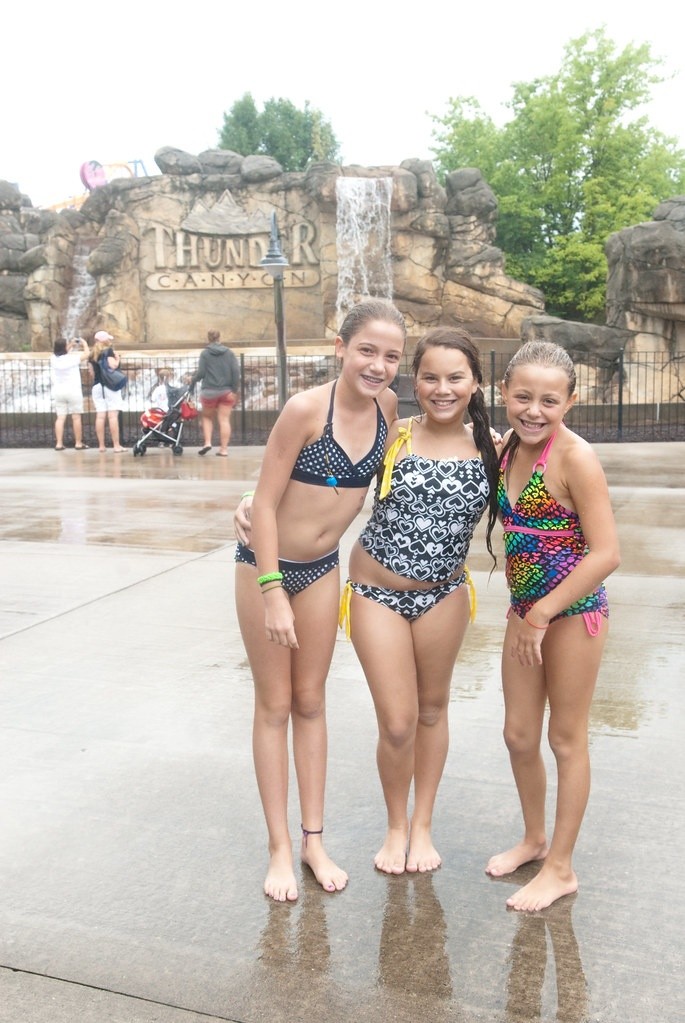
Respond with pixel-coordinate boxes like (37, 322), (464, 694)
(95, 331), (114, 341)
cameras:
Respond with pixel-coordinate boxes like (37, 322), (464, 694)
(75, 339), (80, 344)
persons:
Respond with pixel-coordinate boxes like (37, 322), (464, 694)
(149, 369), (176, 448)
(87, 331), (127, 453)
(50, 337), (91, 450)
(235, 298), (406, 902)
(187, 330), (239, 457)
(233, 328), (501, 875)
(484, 340), (621, 912)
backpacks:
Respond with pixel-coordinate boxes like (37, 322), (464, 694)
(140, 408), (167, 429)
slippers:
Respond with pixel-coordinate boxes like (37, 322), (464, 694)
(216, 451), (228, 456)
(55, 445), (66, 450)
(198, 445), (212, 455)
(75, 442), (89, 449)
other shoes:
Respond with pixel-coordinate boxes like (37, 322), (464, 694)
(114, 447), (128, 453)
(158, 441), (165, 448)
(99, 448), (109, 451)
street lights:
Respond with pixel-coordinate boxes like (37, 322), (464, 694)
(258, 210), (289, 413)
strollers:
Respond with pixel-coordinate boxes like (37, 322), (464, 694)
(132, 376), (194, 455)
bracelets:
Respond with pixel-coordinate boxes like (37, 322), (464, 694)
(257, 572), (283, 585)
(262, 585), (283, 593)
(240, 490), (254, 500)
(525, 613), (549, 629)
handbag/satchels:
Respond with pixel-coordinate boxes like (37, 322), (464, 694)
(181, 401), (198, 419)
(98, 351), (127, 391)
